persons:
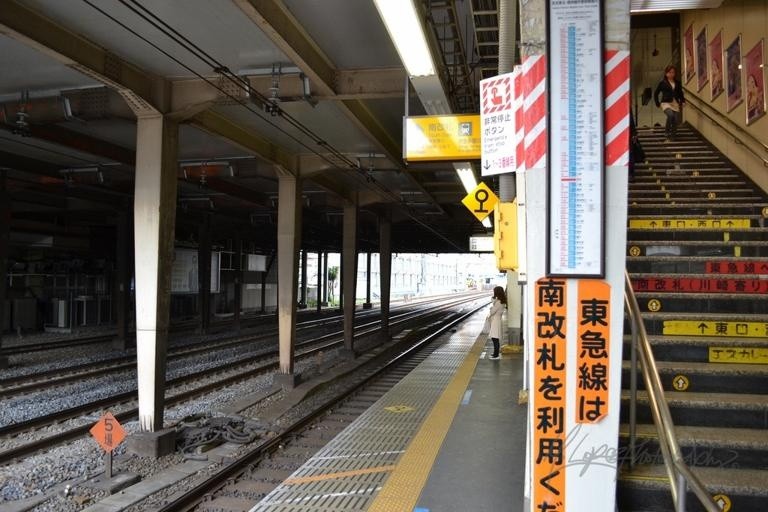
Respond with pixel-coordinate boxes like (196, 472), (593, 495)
(653, 63), (687, 143)
(685, 47), (693, 83)
(710, 56), (723, 98)
(488, 285), (507, 360)
(746, 73), (763, 120)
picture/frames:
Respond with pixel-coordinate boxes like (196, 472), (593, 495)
(683, 20), (767, 127)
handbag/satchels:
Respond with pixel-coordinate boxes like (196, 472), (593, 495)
(482, 316), (491, 334)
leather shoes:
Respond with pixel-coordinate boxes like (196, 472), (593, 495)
(489, 354), (500, 360)
